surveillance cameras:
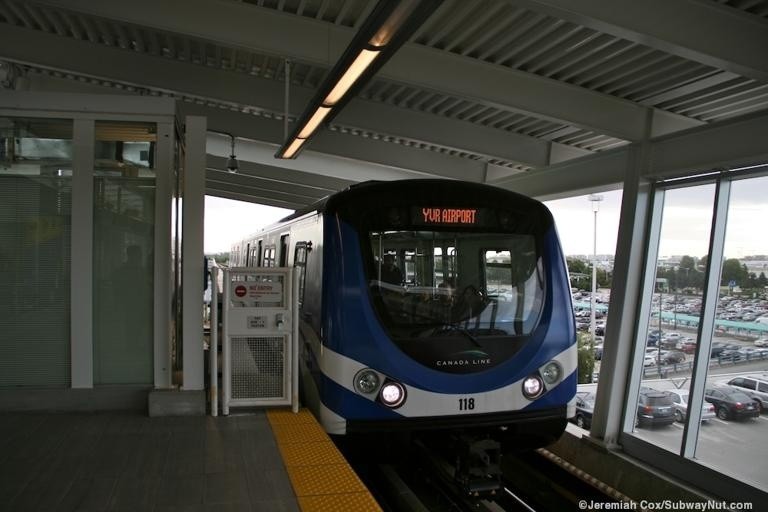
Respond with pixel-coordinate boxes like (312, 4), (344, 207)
(225, 158), (241, 173)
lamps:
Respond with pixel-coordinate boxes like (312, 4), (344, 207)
(274, 0), (446, 159)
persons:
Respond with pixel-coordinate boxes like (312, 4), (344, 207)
(416, 287), (435, 305)
(450, 285), (484, 324)
(101, 245), (153, 382)
(371, 253), (403, 286)
(437, 282), (457, 307)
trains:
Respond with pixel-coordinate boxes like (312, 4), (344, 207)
(227, 179), (578, 454)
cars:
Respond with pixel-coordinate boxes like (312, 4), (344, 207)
(570, 374), (768, 428)
(571, 288), (767, 367)
